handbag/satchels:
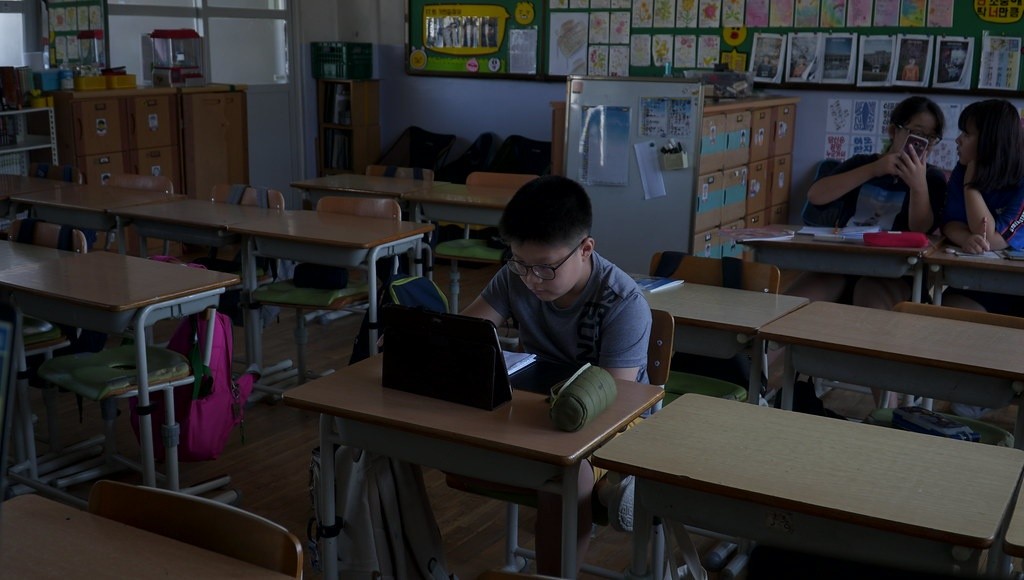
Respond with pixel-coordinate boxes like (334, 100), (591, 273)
(657, 513), (708, 580)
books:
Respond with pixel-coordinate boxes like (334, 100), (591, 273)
(948, 245), (1024, 261)
(0, 209), (29, 240)
(310, 41), (374, 80)
(0, 151), (29, 195)
(323, 128), (350, 172)
(720, 224), (881, 241)
(0, 65), (36, 112)
(324, 82), (351, 124)
(0, 113), (29, 149)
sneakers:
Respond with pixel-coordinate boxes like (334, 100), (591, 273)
(608, 475), (634, 532)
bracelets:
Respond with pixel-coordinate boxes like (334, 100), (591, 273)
(963, 183), (970, 187)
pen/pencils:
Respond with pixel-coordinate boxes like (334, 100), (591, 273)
(982, 216), (987, 255)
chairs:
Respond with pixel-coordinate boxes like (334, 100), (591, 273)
(0, 166), (1024, 580)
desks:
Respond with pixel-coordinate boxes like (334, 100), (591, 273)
(0, 240), (82, 275)
(624, 269), (807, 404)
(291, 173), (449, 276)
(229, 210), (436, 376)
(593, 393), (1024, 580)
(745, 225), (940, 318)
(756, 300), (1024, 413)
(0, 173), (77, 231)
(1, 252), (241, 494)
(9, 184), (175, 268)
(928, 242), (1024, 317)
(107, 196), (258, 296)
(281, 351), (665, 580)
(404, 183), (519, 290)
(0, 494), (297, 580)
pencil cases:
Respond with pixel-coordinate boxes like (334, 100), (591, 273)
(543, 361), (620, 435)
(862, 230), (930, 249)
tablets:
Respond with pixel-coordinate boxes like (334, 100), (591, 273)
(383, 302), (513, 413)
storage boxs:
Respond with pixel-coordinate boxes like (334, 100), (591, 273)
(150, 29), (201, 68)
(311, 41), (374, 79)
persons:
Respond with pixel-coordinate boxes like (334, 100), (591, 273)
(97, 119), (107, 135)
(902, 56), (919, 81)
(807, 96), (950, 409)
(793, 55), (808, 76)
(945, 99), (1024, 419)
(756, 55), (777, 79)
(102, 175), (110, 185)
(455, 177), (653, 579)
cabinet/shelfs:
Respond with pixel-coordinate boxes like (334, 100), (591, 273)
(46, 87), (185, 256)
(549, 93), (800, 265)
(316, 80), (381, 176)
(180, 82), (249, 202)
(0, 107), (57, 231)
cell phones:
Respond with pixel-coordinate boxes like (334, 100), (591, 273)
(896, 135), (928, 170)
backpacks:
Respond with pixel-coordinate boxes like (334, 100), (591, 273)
(349, 267), (450, 368)
(375, 164), (434, 278)
(893, 403), (982, 442)
(16, 218), (107, 391)
(773, 369), (848, 420)
(34, 160), (97, 250)
(209, 184), (296, 329)
(338, 445), (457, 580)
(652, 251), (772, 396)
(128, 254), (262, 464)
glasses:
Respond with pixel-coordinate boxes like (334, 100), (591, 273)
(894, 122), (944, 147)
(506, 237), (595, 280)
